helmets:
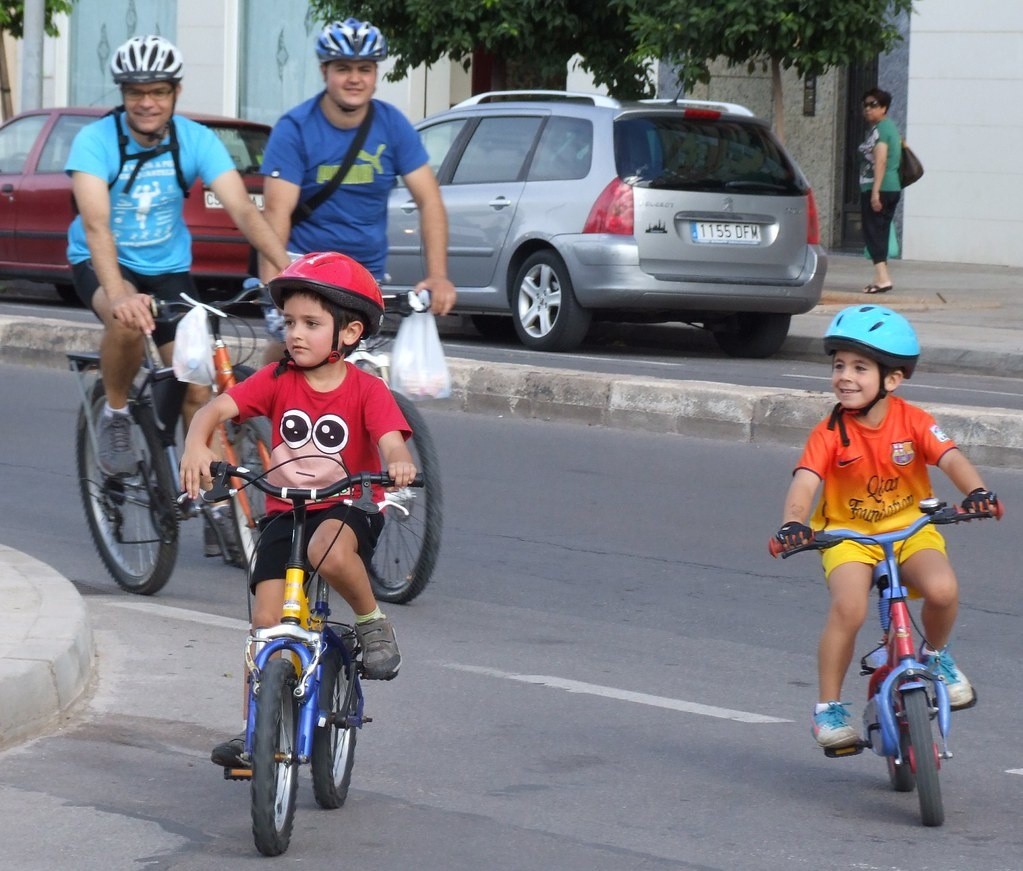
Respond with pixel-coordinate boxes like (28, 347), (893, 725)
(267, 251), (386, 340)
(823, 303), (920, 379)
(315, 17), (387, 63)
(109, 34), (184, 84)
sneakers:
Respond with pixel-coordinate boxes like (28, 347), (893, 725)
(809, 699), (858, 749)
(354, 616), (402, 681)
(921, 644), (972, 708)
(209, 728), (247, 767)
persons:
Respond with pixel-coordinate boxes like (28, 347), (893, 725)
(180, 251), (416, 769)
(257, 18), (457, 367)
(66, 36), (304, 477)
(771, 305), (1004, 748)
(858, 88), (902, 294)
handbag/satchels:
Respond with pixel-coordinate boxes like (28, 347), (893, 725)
(388, 291), (452, 403)
(173, 291), (227, 387)
(898, 146), (924, 186)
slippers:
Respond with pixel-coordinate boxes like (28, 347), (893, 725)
(863, 284), (892, 293)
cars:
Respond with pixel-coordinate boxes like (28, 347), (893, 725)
(0, 106), (270, 298)
(284, 88), (825, 357)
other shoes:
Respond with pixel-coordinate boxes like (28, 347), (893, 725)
(94, 407), (139, 479)
(203, 506), (240, 556)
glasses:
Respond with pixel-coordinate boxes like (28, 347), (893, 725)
(124, 86), (173, 101)
(861, 100), (880, 109)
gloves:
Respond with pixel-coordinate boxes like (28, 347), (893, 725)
(960, 487), (997, 515)
(776, 521), (813, 551)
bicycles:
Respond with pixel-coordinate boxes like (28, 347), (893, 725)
(770, 486), (1003, 827)
(71, 276), (281, 592)
(177, 460), (429, 859)
(238, 275), (445, 609)
